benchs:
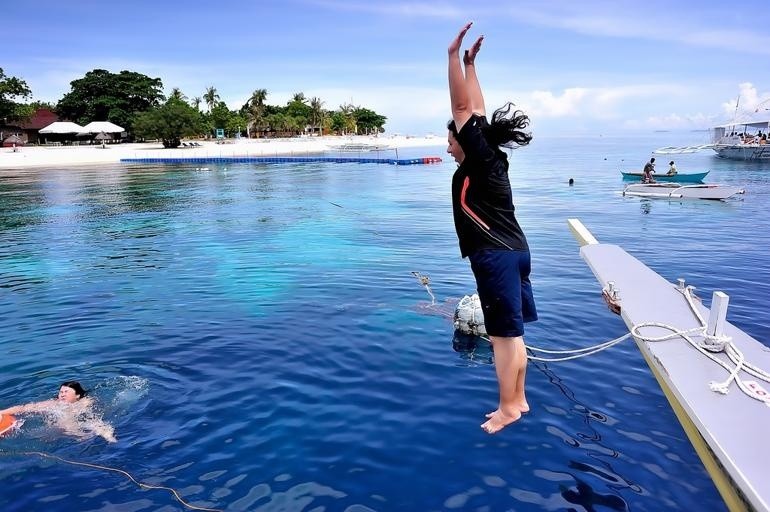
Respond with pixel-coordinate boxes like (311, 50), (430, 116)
(720, 135), (745, 144)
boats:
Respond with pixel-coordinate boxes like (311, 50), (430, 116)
(622, 178), (746, 201)
(621, 170), (712, 185)
(651, 96), (770, 162)
(562, 215), (770, 512)
(328, 143), (389, 153)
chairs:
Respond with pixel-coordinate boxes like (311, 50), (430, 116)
(37, 137), (92, 147)
(182, 140), (202, 149)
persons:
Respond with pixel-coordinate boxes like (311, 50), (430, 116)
(667, 160), (678, 174)
(641, 167), (656, 184)
(446, 21), (539, 434)
(643, 157), (657, 172)
(761, 134), (766, 140)
(756, 130), (762, 136)
(0, 381), (101, 441)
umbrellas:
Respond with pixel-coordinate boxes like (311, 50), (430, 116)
(38, 121), (85, 134)
(95, 131), (112, 148)
(75, 133), (92, 137)
(82, 121), (125, 134)
